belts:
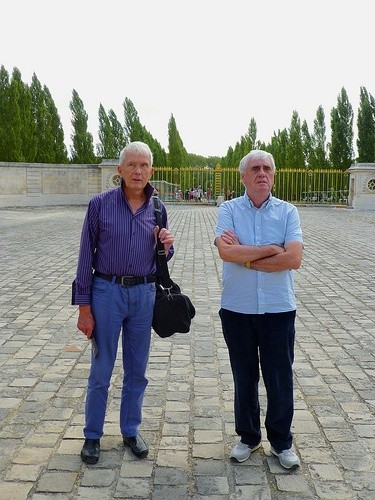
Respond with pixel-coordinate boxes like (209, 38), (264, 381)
(94, 271), (157, 287)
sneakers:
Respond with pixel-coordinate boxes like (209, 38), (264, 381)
(230, 440), (263, 463)
(269, 444), (301, 468)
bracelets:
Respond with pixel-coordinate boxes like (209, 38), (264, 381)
(245, 262), (250, 267)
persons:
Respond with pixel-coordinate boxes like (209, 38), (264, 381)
(154, 189), (158, 196)
(221, 186), (231, 200)
(212, 149), (303, 469)
(179, 185), (213, 202)
(71, 141), (175, 464)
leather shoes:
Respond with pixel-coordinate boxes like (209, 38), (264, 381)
(80, 438), (101, 464)
(123, 435), (148, 458)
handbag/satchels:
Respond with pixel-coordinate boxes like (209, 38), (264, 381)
(150, 278), (192, 339)
